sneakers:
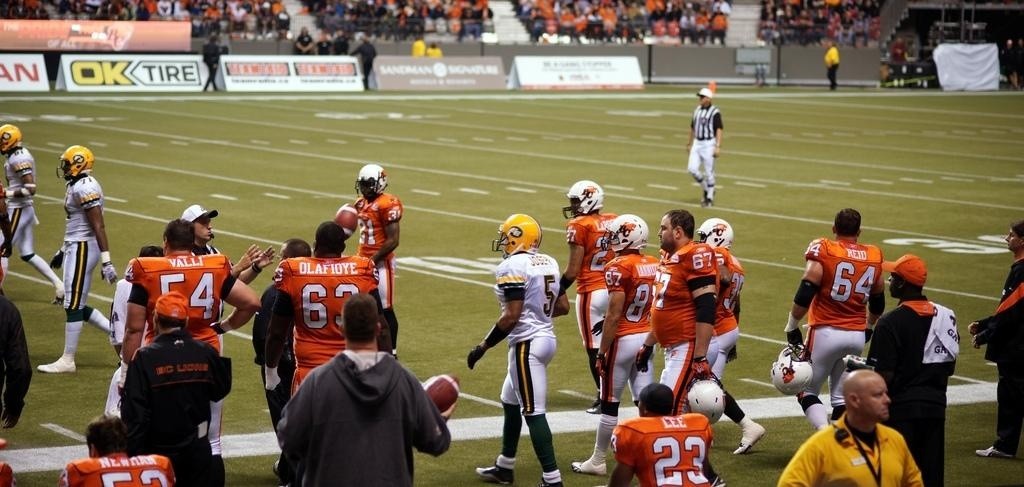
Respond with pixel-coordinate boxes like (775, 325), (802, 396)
(476, 461), (516, 486)
(732, 418), (767, 457)
(700, 191), (708, 204)
(975, 447), (1015, 460)
(571, 459), (610, 477)
(537, 474), (564, 487)
(36, 354), (78, 375)
(51, 285), (66, 306)
(586, 402), (602, 415)
(702, 198), (713, 208)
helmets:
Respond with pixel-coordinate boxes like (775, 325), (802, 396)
(55, 144), (95, 182)
(490, 213), (545, 260)
(696, 217), (735, 253)
(770, 344), (814, 397)
(561, 179), (606, 222)
(600, 213), (652, 254)
(685, 374), (728, 426)
(0, 124), (24, 156)
(354, 163), (390, 202)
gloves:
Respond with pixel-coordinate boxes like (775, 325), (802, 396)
(690, 356), (712, 381)
(101, 260), (118, 286)
(635, 343), (655, 374)
(595, 354), (608, 378)
(865, 328), (873, 343)
(590, 315), (606, 337)
(465, 343), (487, 372)
(49, 249), (66, 271)
(785, 327), (804, 347)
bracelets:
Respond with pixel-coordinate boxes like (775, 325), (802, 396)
(560, 273), (573, 290)
(692, 355), (708, 363)
(793, 279), (819, 310)
(6, 191), (14, 198)
(21, 187), (31, 197)
(868, 291), (885, 315)
(715, 144), (721, 148)
(219, 317), (232, 332)
(251, 262), (261, 274)
(597, 354), (605, 360)
(484, 323), (506, 348)
(687, 143), (691, 146)
(693, 292), (717, 326)
(784, 311), (799, 333)
(98, 251), (113, 264)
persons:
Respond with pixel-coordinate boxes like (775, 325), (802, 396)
(299, 0), (351, 35)
(180, 203), (276, 487)
(1, 0), (293, 41)
(294, 27), (314, 55)
(686, 87), (723, 208)
(967, 219), (1024, 460)
(604, 382), (714, 487)
(757, 0), (883, 46)
(118, 218), (262, 398)
(119, 290), (234, 486)
(570, 213), (660, 476)
(58, 414), (178, 486)
(999, 37), (1024, 92)
(349, 0), (495, 40)
(412, 36), (427, 56)
(776, 369), (924, 487)
(427, 42), (443, 58)
(694, 216), (766, 456)
(353, 162), (403, 360)
(843, 253), (961, 486)
(466, 213), (571, 487)
(104, 245), (164, 421)
(36, 143), (115, 373)
(334, 28), (348, 56)
(635, 209), (726, 487)
(202, 33), (220, 93)
(783, 207), (886, 431)
(264, 220), (393, 401)
(888, 35), (908, 87)
(251, 237), (314, 486)
(559, 179), (620, 415)
(349, 35), (376, 90)
(0, 261), (33, 447)
(0, 123), (66, 303)
(824, 41), (840, 91)
(275, 292), (461, 487)
(509, 0), (735, 46)
(0, 182), (13, 258)
(316, 33), (332, 56)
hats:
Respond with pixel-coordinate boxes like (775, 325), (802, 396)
(696, 88), (715, 100)
(181, 204), (220, 223)
(880, 252), (929, 291)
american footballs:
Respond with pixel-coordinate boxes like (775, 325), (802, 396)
(334, 203), (358, 241)
(421, 374), (461, 422)
